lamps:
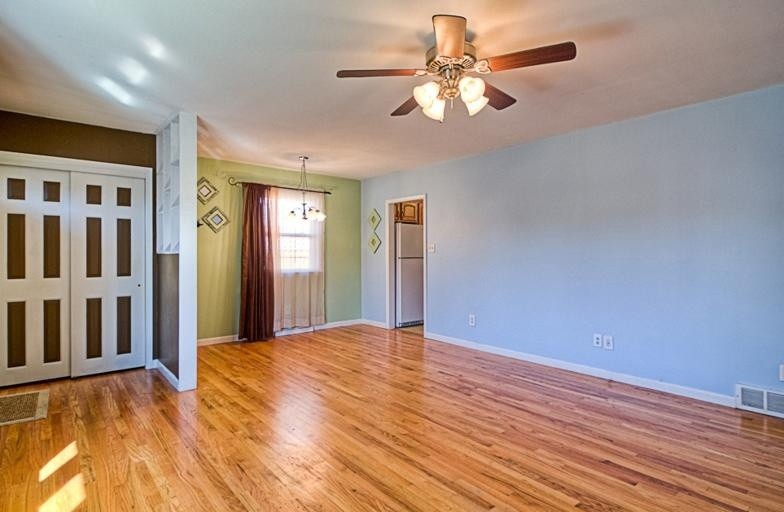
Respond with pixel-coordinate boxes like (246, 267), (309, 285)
(289, 155), (327, 222)
(414, 76), (490, 122)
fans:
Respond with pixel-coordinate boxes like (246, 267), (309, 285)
(336, 14), (577, 117)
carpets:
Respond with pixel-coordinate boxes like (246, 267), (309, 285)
(0, 388), (50, 426)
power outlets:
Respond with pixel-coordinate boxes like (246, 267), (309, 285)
(469, 315), (475, 327)
(593, 334), (613, 350)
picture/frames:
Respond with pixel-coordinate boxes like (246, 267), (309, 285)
(197, 177), (231, 234)
(367, 207), (382, 254)
